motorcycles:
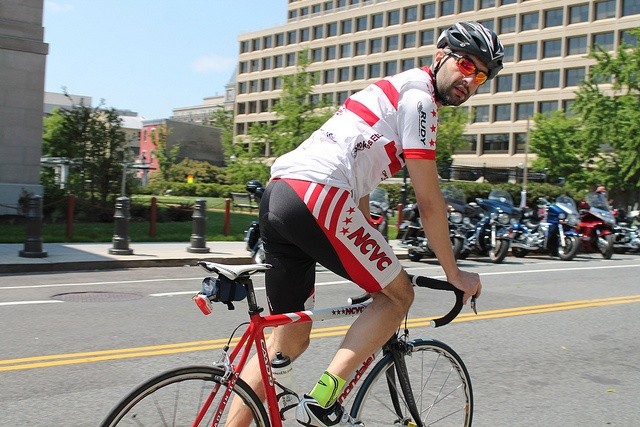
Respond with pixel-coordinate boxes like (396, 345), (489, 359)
(366, 188), (389, 242)
(571, 183), (620, 259)
(612, 207), (639, 253)
(473, 189), (581, 260)
(398, 189), (520, 260)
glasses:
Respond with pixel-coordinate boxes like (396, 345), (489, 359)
(447, 51), (487, 88)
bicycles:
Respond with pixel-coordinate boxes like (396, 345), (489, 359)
(97, 260), (478, 427)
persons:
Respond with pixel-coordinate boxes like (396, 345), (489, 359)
(222, 21), (504, 427)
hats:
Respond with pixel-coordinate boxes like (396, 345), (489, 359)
(595, 186), (607, 193)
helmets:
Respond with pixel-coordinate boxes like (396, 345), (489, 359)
(436, 20), (506, 81)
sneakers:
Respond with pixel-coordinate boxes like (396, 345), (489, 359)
(296, 394), (363, 427)
